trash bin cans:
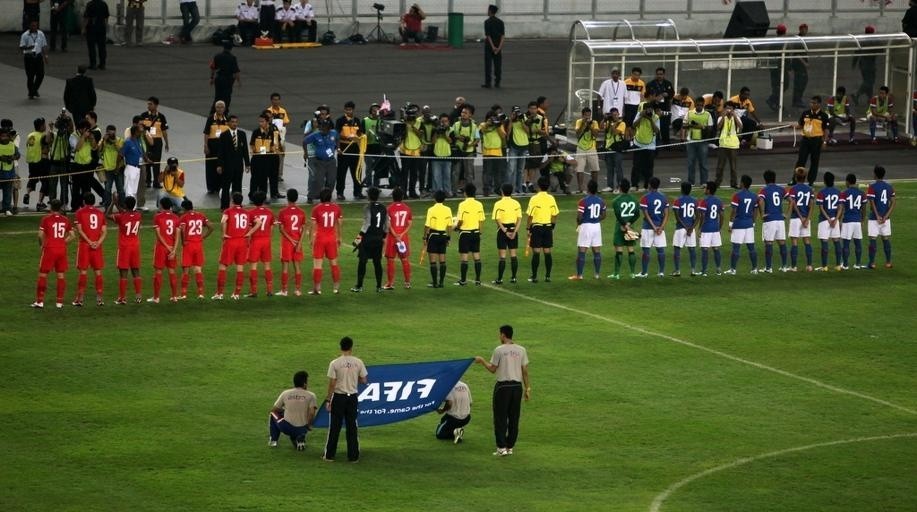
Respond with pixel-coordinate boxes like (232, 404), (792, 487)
(448, 13), (464, 48)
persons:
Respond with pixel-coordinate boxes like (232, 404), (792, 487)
(321, 336), (369, 464)
(267, 370), (318, 452)
(474, 325), (532, 457)
(434, 379), (472, 444)
(2, 0), (917, 218)
(31, 166), (895, 308)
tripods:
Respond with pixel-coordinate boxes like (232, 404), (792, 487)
(366, 12), (391, 43)
(40, 131), (74, 216)
(360, 149), (408, 198)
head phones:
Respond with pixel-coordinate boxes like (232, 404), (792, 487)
(369, 103), (381, 114)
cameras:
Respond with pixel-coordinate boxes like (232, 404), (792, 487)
(87, 128), (100, 136)
(527, 115), (540, 124)
(691, 120), (698, 126)
(493, 112), (506, 126)
(462, 135), (470, 150)
(605, 113), (612, 122)
(432, 124), (446, 134)
(646, 108), (652, 117)
(430, 115), (438, 122)
(109, 133), (115, 140)
(314, 110), (332, 127)
(10, 128), (17, 137)
(5, 155), (13, 164)
(554, 152), (560, 158)
(143, 124), (150, 130)
(170, 165), (177, 171)
(586, 118), (593, 125)
(513, 106), (523, 119)
(725, 109), (733, 116)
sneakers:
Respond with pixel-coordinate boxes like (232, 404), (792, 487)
(493, 447), (515, 456)
(1, 181), (163, 217)
(827, 135), (901, 144)
(296, 439), (306, 452)
(250, 193), (286, 204)
(454, 428), (464, 443)
(267, 436), (277, 448)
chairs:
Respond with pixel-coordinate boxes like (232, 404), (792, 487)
(575, 88), (603, 112)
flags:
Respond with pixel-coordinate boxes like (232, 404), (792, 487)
(311, 357), (477, 429)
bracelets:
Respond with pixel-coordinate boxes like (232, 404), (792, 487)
(524, 386), (531, 393)
(326, 398), (330, 403)
